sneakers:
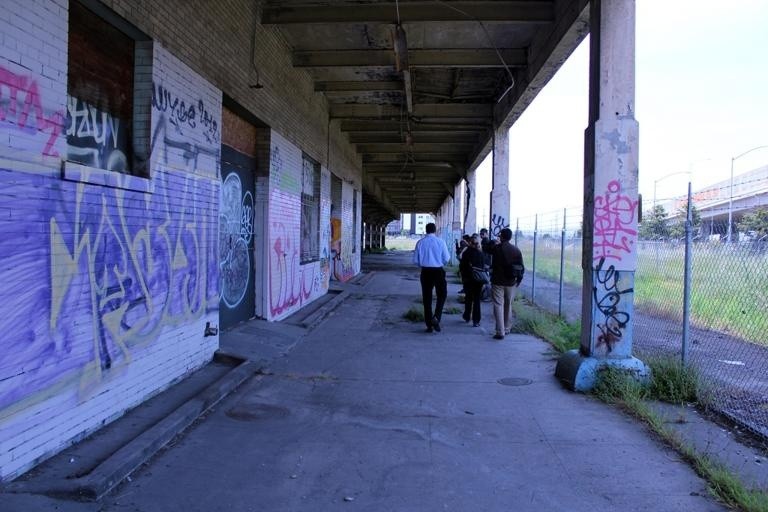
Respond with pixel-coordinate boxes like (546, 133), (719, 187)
(492, 330), (511, 340)
(462, 312), (481, 327)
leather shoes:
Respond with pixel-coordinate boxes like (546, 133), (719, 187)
(425, 316), (441, 333)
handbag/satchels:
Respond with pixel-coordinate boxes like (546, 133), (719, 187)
(472, 266), (490, 284)
(512, 263), (522, 276)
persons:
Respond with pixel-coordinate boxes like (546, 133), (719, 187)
(453, 227), (501, 302)
(458, 237), (487, 328)
(414, 222), (451, 333)
(486, 228), (524, 340)
